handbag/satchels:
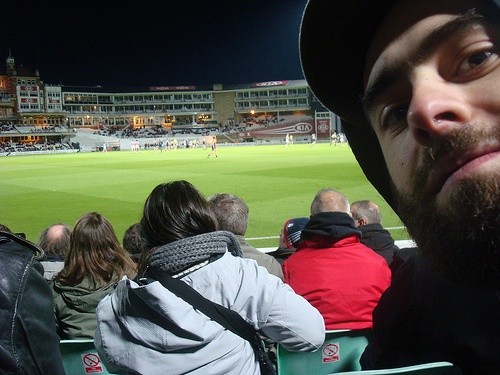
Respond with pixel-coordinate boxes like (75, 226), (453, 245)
(253, 334), (277, 374)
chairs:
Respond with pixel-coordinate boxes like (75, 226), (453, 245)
(276, 330), (369, 375)
(324, 362), (453, 375)
(60, 340), (119, 375)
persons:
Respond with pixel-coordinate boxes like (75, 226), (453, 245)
(264, 217), (310, 264)
(95, 179), (326, 375)
(48, 211), (138, 342)
(0, 115), (81, 153)
(285, 131), (348, 146)
(38, 224), (72, 256)
(83, 116), (285, 140)
(1, 223), (66, 375)
(123, 221), (144, 255)
(350, 199), (402, 272)
(280, 188), (392, 331)
(207, 192), (285, 282)
(208, 137), (217, 158)
(298, 0), (500, 373)
(101, 136), (216, 153)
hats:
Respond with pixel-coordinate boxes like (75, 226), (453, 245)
(287, 218), (310, 247)
(298, 1), (398, 129)
